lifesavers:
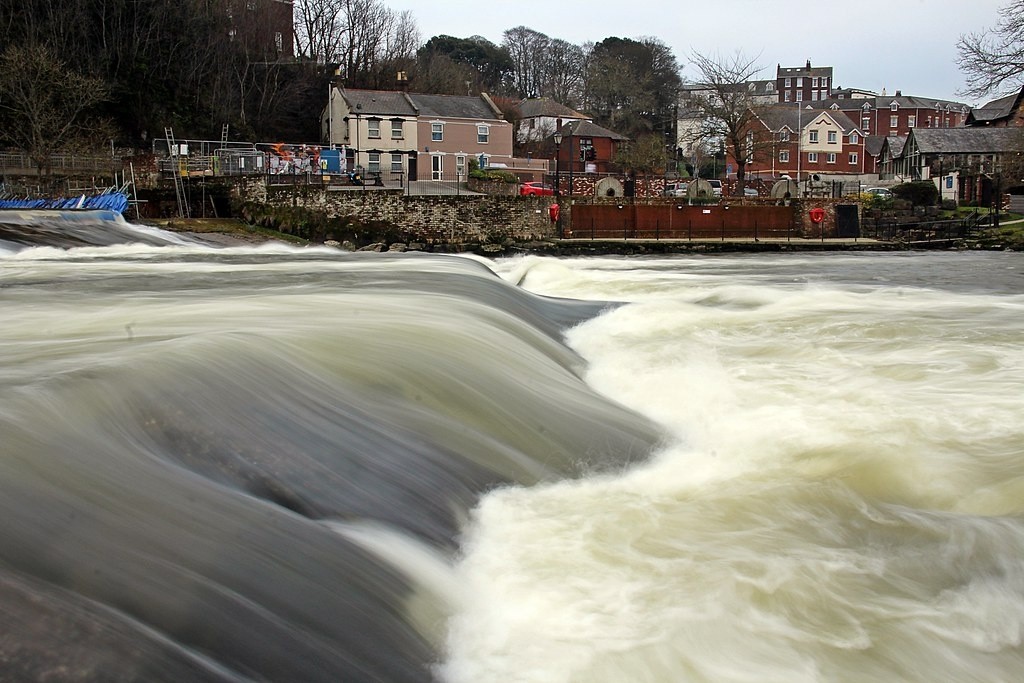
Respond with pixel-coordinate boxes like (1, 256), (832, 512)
(810, 208), (826, 223)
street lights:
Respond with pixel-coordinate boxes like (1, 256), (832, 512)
(324, 61), (341, 150)
(710, 153), (716, 180)
(553, 131), (562, 196)
(937, 155), (944, 205)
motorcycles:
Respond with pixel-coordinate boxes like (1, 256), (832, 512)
(341, 169), (364, 186)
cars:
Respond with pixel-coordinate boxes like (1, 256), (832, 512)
(856, 187), (893, 200)
(708, 179), (724, 198)
(516, 180), (554, 196)
(658, 183), (687, 196)
(744, 188), (759, 197)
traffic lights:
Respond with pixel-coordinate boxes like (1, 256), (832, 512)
(579, 148), (585, 162)
(587, 147), (597, 161)
